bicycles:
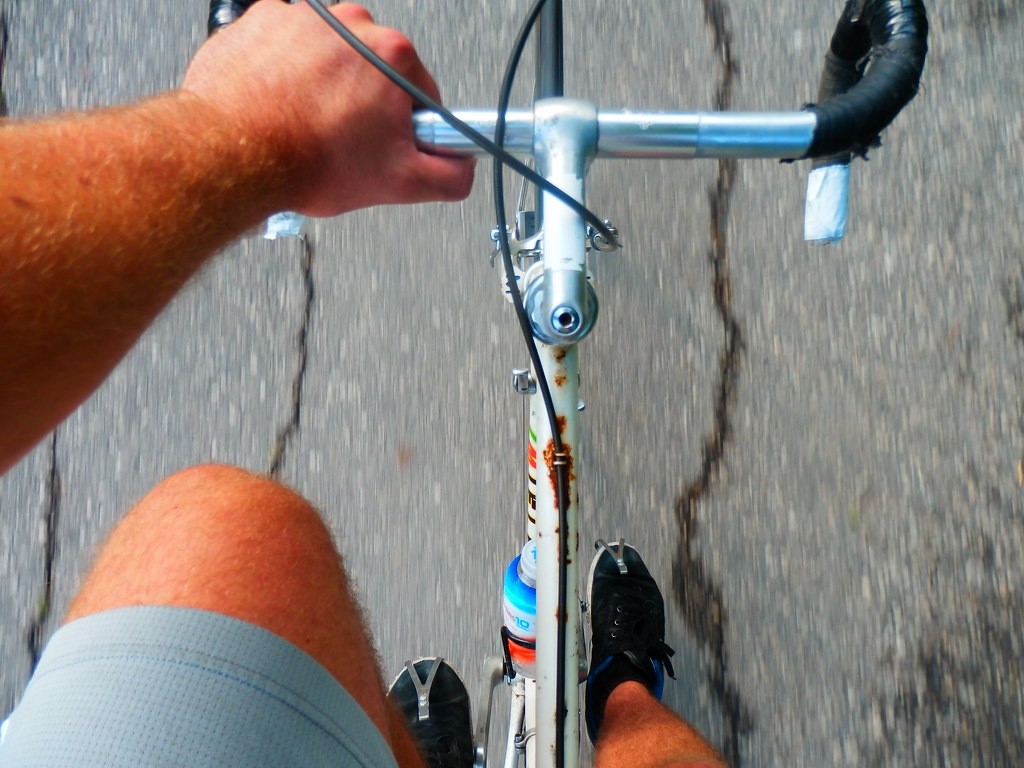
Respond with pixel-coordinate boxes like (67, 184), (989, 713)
(196, 0), (930, 768)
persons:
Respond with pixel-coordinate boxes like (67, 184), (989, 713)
(0, 0), (733, 768)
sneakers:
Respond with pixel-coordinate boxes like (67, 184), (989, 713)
(385, 656), (474, 768)
(584, 542), (676, 748)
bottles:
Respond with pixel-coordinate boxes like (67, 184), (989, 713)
(503, 540), (538, 678)
